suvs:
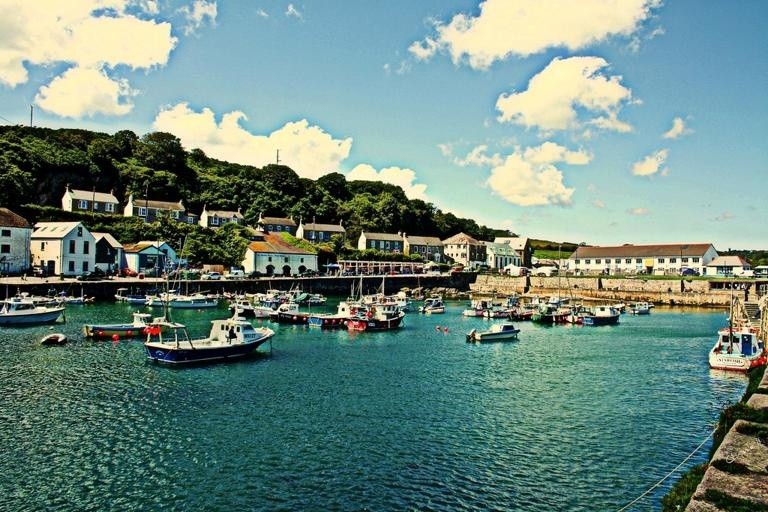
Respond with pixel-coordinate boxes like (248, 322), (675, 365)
(447, 264), (490, 277)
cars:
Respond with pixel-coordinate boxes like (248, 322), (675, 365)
(680, 268), (704, 277)
(76, 271), (102, 281)
(33, 267), (45, 277)
(297, 268), (321, 278)
(339, 268), (412, 276)
(118, 267), (139, 278)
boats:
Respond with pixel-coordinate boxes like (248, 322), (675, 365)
(160, 268), (260, 281)
(707, 281), (764, 371)
(707, 366), (749, 420)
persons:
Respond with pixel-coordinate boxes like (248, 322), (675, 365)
(228, 325), (237, 340)
(742, 285), (750, 301)
(752, 301), (766, 319)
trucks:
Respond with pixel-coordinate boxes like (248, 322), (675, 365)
(733, 268), (767, 278)
